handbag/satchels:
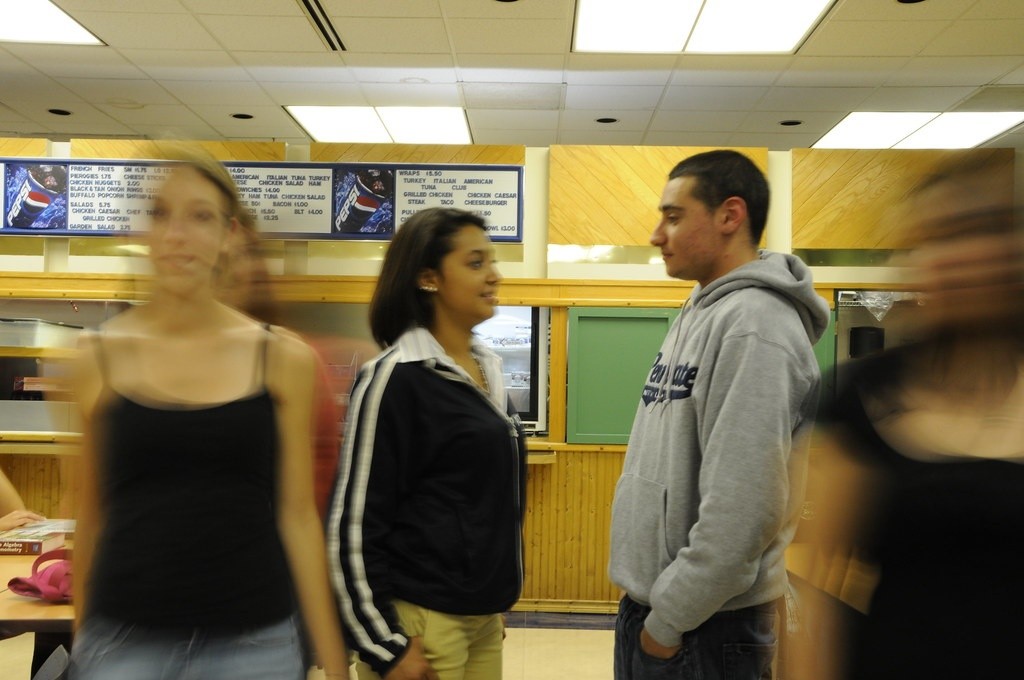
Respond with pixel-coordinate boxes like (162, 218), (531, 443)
(6, 548), (75, 601)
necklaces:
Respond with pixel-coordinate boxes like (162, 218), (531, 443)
(474, 357), (490, 395)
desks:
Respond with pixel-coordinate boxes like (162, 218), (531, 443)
(0, 538), (78, 634)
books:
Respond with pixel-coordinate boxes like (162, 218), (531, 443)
(0, 529), (67, 555)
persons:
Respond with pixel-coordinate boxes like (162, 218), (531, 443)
(322, 207), (526, 680)
(790, 151), (1024, 680)
(67, 154), (350, 680)
(0, 469), (48, 532)
(600, 149), (830, 680)
